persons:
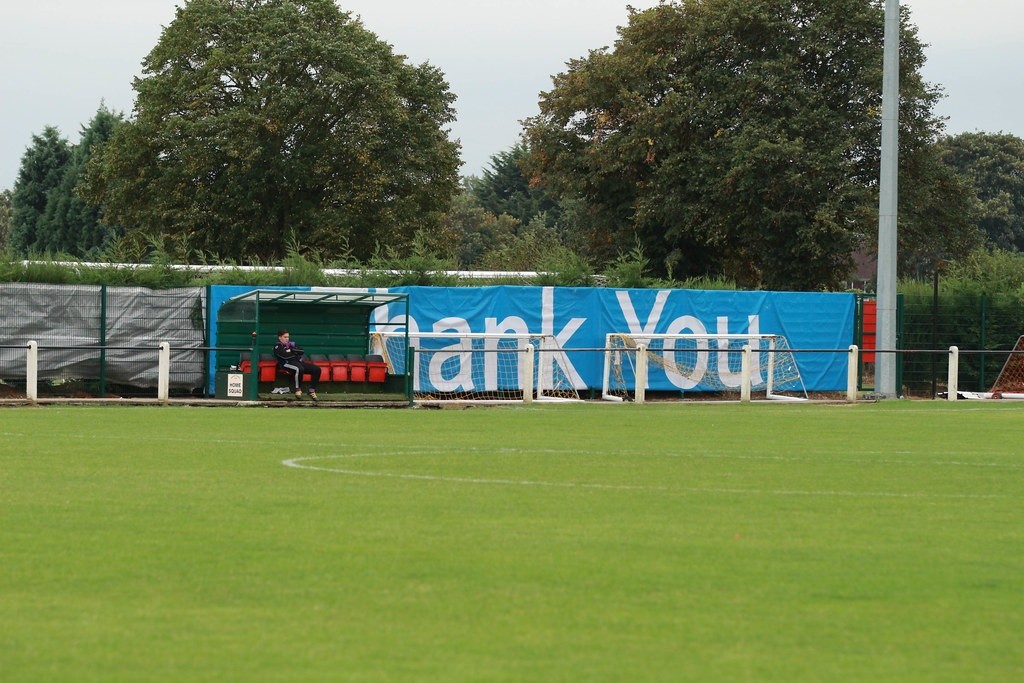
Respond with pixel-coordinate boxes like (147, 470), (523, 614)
(273, 329), (321, 403)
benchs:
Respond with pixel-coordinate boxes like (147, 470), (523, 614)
(241, 353), (388, 383)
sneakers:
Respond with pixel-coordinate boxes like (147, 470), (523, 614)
(307, 387), (318, 401)
(294, 387), (302, 399)
